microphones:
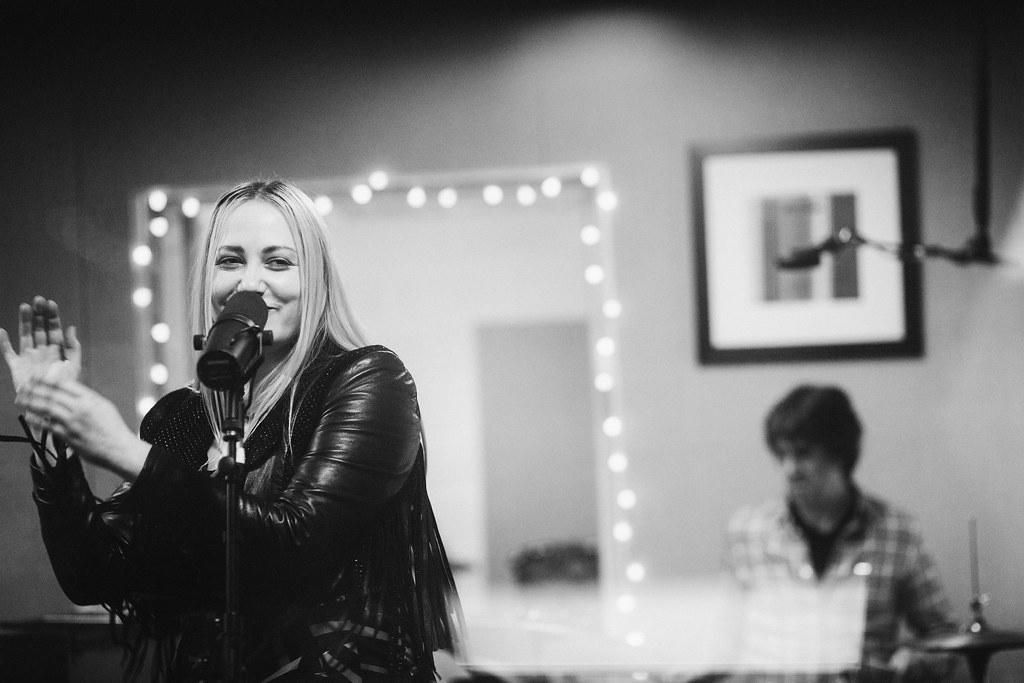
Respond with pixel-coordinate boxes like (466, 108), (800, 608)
(195, 290), (269, 391)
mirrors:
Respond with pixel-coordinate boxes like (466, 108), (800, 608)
(125, 164), (629, 610)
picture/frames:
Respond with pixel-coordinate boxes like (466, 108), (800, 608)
(684, 127), (927, 366)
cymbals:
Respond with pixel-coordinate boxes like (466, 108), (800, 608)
(899, 623), (1024, 654)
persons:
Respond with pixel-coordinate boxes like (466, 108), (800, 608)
(0, 180), (464, 683)
(727, 384), (958, 683)
